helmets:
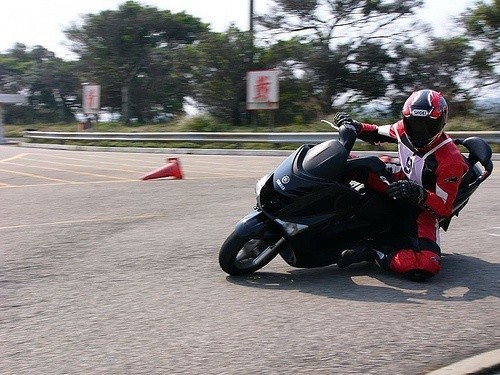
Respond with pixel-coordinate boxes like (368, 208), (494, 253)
(402, 89), (449, 151)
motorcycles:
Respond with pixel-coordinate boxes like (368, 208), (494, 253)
(218, 120), (493, 276)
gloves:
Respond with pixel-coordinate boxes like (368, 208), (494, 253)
(333, 112), (361, 133)
(386, 180), (427, 205)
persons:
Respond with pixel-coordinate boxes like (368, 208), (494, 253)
(333, 87), (467, 283)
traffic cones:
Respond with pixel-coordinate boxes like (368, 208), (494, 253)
(140, 157), (185, 181)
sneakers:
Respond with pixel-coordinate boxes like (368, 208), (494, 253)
(337, 242), (370, 269)
